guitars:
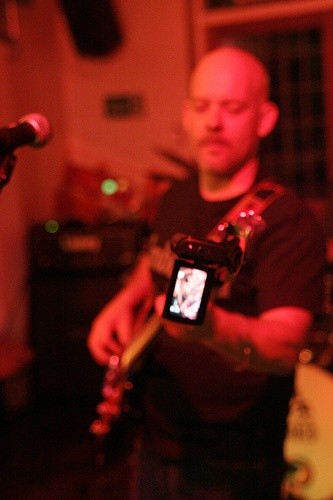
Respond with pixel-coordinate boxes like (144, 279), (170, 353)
(90, 206), (266, 462)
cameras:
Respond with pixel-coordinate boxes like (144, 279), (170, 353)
(160, 236), (219, 328)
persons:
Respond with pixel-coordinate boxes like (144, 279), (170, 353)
(85, 46), (328, 500)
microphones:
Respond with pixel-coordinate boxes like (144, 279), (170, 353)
(0, 113), (52, 160)
